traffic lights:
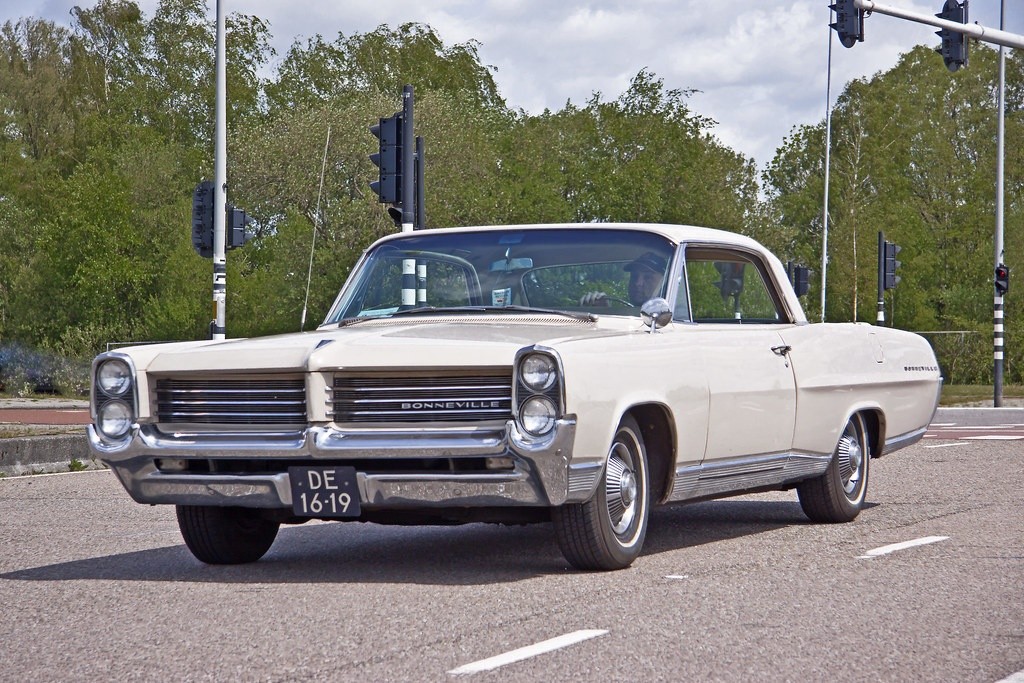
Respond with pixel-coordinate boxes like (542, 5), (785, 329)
(934, 0), (969, 72)
(884, 242), (903, 289)
(713, 262), (744, 302)
(387, 207), (401, 226)
(995, 263), (1010, 295)
(368, 113), (401, 204)
(229, 204), (254, 248)
(827, 0), (864, 49)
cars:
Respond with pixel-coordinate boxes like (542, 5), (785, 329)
(88, 220), (944, 573)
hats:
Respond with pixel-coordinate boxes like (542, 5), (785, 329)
(624, 251), (668, 274)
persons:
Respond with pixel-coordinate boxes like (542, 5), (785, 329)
(579, 252), (666, 306)
